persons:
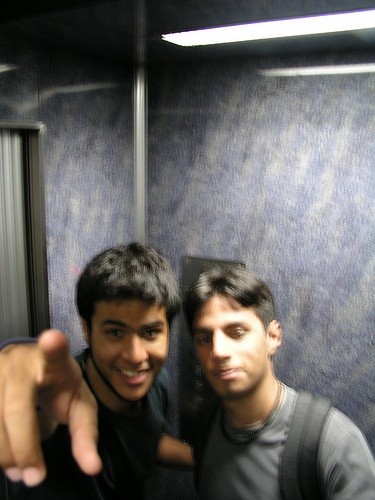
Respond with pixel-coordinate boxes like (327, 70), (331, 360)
(1, 242), (193, 500)
(183, 265), (375, 500)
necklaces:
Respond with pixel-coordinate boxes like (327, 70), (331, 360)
(220, 379), (281, 445)
